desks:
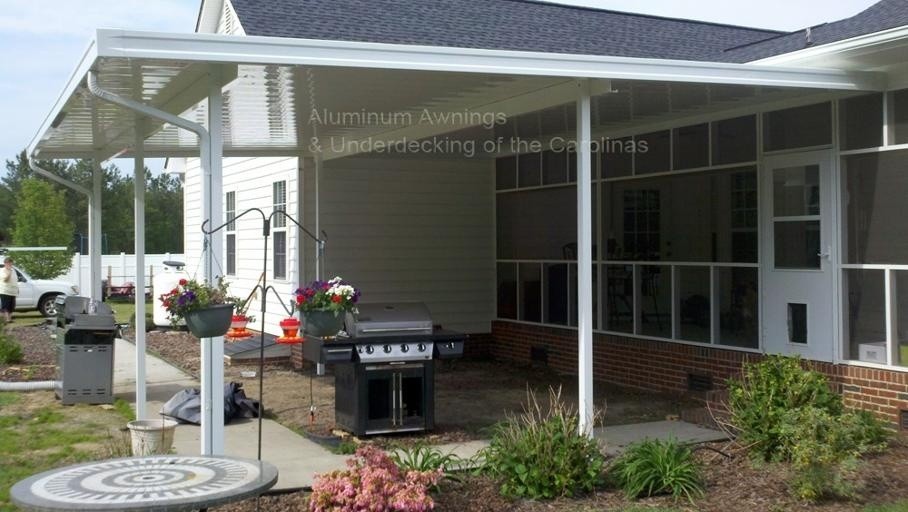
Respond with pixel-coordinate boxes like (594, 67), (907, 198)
(7, 456), (279, 512)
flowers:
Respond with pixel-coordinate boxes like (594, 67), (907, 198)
(158, 276), (248, 330)
(292, 276), (362, 318)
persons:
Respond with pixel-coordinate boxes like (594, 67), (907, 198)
(0, 256), (20, 323)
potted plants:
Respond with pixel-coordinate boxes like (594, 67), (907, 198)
(228, 297), (255, 338)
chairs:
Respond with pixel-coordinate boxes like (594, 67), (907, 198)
(562, 242), (577, 260)
(607, 239), (668, 331)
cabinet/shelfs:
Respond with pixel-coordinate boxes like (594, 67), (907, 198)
(104, 265), (153, 299)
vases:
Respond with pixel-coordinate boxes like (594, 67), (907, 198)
(299, 307), (348, 336)
(183, 302), (236, 338)
(273, 317), (306, 345)
(127, 418), (178, 457)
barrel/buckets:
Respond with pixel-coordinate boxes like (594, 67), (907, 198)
(127, 400), (178, 457)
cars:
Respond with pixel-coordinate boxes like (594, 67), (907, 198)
(0, 264), (80, 317)
